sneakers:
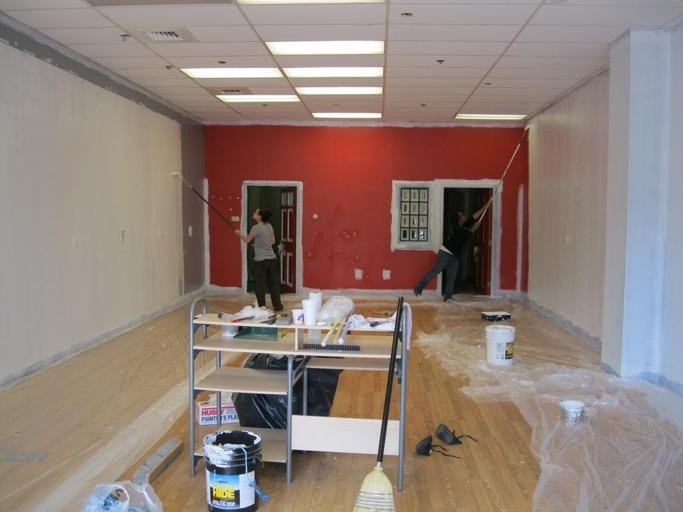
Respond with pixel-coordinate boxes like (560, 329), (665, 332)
(415, 423), (463, 456)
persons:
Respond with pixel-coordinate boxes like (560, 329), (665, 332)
(232, 208), (284, 311)
(413, 200), (491, 304)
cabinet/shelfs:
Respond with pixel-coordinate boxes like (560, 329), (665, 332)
(189, 297), (412, 492)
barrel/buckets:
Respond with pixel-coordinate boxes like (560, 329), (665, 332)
(203, 429), (269, 511)
(485, 313), (517, 367)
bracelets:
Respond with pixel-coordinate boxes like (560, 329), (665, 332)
(481, 206), (486, 212)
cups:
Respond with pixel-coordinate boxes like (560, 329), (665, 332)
(309, 289), (322, 312)
(302, 299), (318, 326)
(293, 309), (304, 324)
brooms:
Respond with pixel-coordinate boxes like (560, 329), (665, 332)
(352, 297), (404, 511)
